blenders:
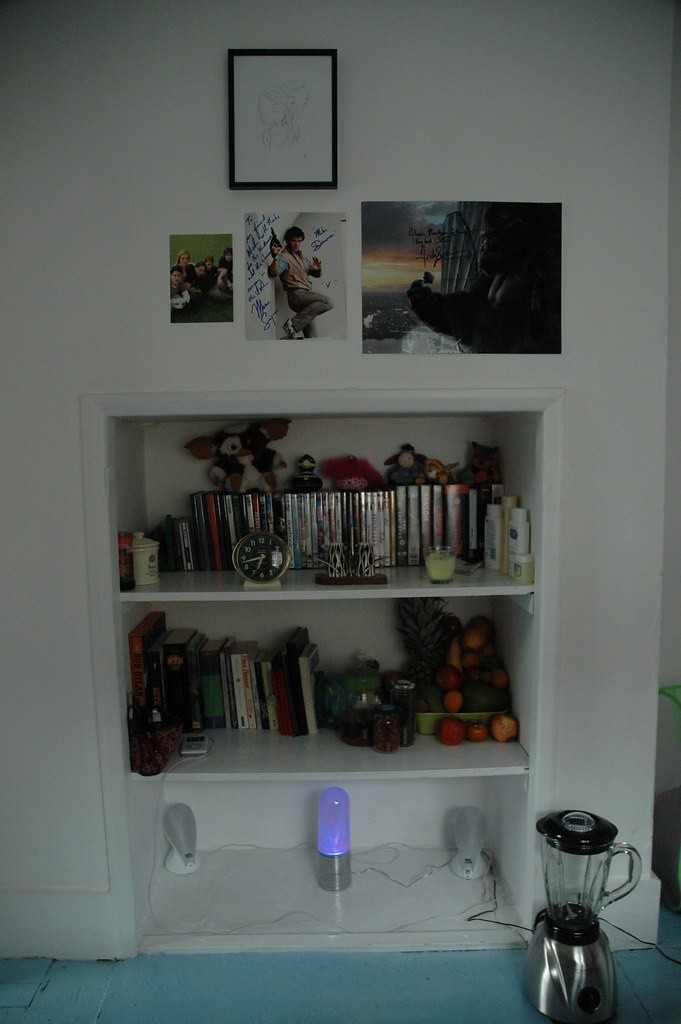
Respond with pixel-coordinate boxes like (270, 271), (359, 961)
(521, 809), (642, 1024)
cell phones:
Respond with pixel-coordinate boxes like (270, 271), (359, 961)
(180, 733), (209, 758)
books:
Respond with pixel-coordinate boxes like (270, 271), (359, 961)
(129, 611), (322, 736)
(173, 482), (491, 572)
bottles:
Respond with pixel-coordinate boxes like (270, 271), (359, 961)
(484, 503), (504, 570)
(508, 508), (530, 577)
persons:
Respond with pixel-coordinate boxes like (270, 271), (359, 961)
(268, 227), (334, 339)
(171, 248), (233, 318)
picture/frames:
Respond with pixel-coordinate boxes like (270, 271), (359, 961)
(227, 48), (340, 192)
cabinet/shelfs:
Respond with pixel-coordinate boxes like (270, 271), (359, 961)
(78, 387), (569, 953)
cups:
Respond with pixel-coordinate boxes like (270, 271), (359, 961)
(127, 531), (161, 586)
(422, 545), (457, 584)
